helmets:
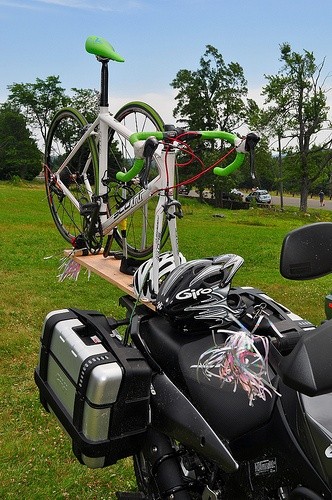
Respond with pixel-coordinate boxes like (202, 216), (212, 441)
(133, 249), (185, 302)
(156, 254), (247, 335)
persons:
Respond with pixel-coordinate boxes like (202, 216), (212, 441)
(319, 190), (324, 207)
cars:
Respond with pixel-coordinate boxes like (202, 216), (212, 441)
(245, 190), (272, 204)
(178, 184), (243, 200)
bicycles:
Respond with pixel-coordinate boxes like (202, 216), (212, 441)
(44, 36), (261, 262)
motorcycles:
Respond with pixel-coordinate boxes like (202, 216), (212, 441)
(33, 222), (332, 500)
